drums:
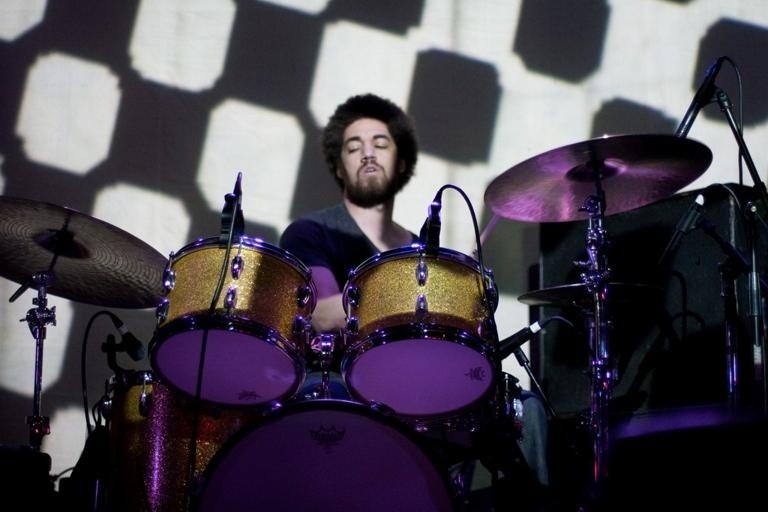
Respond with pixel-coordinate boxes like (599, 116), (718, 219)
(113, 370), (237, 493)
(149, 234), (318, 411)
(191, 399), (461, 510)
(342, 244), (501, 431)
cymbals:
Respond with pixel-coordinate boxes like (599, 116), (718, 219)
(517, 282), (653, 306)
(485, 134), (712, 223)
(2, 200), (167, 309)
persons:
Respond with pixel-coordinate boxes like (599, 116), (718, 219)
(279, 94), (420, 332)
